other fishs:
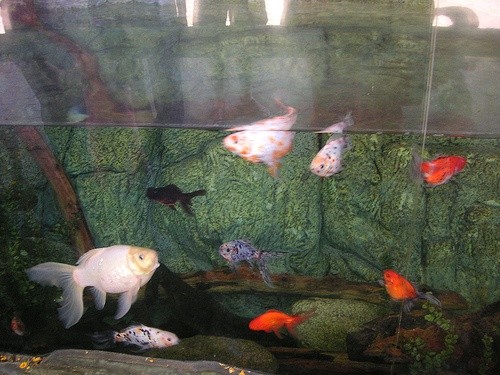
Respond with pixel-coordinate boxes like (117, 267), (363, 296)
(377, 270), (443, 313)
(83, 322), (181, 352)
(407, 146), (467, 187)
(218, 235), (292, 290)
(247, 308), (317, 340)
(221, 130), (296, 182)
(11, 313), (32, 337)
(309, 134), (356, 179)
(24, 244), (162, 329)
(311, 110), (354, 135)
(226, 95), (298, 132)
(146, 184), (208, 222)
